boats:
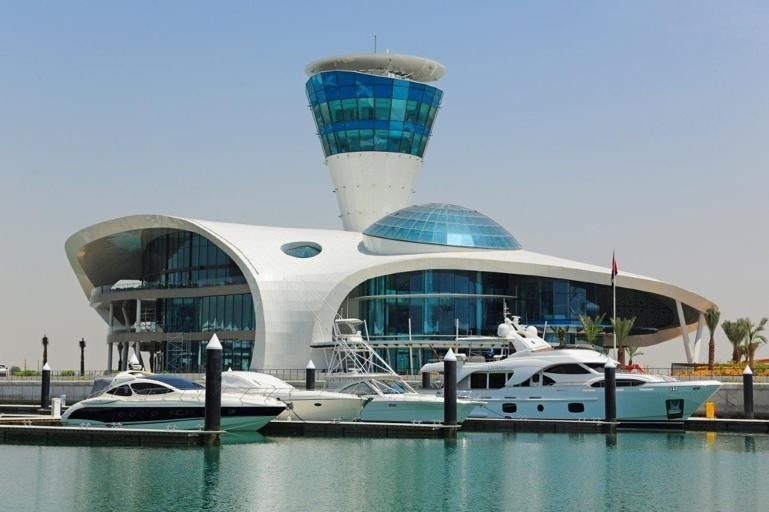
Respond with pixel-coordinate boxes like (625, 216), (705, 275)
(440, 315), (724, 422)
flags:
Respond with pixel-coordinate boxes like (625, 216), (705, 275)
(611, 254), (618, 286)
